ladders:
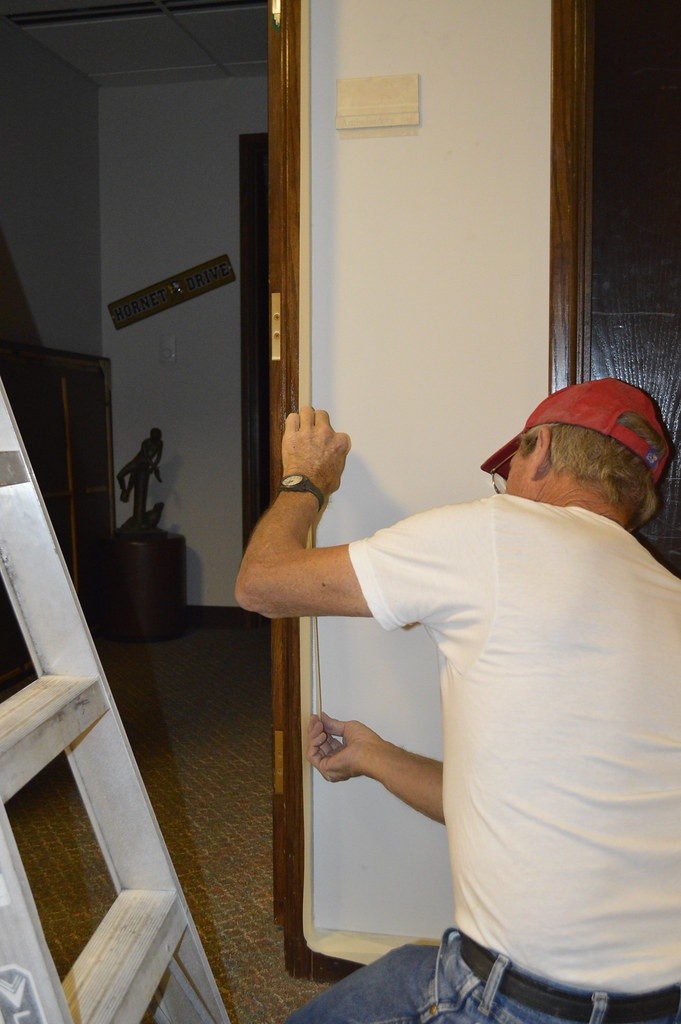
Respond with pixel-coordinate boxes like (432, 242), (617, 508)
(0, 374), (228, 1024)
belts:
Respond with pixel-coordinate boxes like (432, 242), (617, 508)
(460, 933), (681, 1024)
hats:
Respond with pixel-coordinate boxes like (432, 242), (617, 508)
(480, 378), (670, 485)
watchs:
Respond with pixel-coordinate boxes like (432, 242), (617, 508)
(276, 474), (324, 512)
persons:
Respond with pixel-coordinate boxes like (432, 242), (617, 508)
(117, 428), (164, 504)
(233, 378), (681, 1024)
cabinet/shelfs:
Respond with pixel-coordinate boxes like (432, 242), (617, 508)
(0, 341), (116, 689)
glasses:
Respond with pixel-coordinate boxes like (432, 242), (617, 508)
(490, 448), (522, 494)
